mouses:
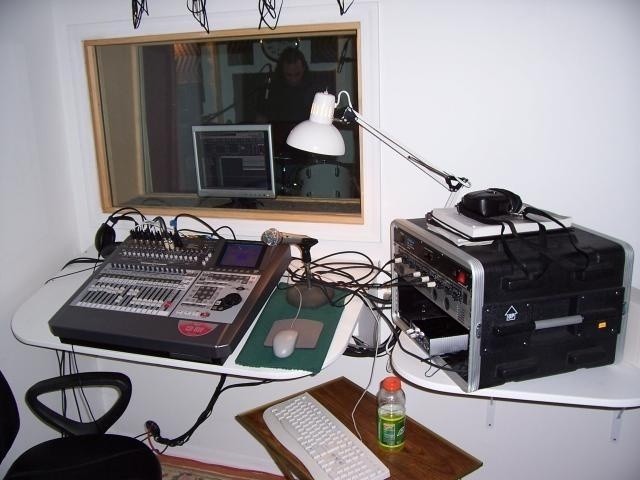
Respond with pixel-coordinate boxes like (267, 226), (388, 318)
(273, 329), (300, 359)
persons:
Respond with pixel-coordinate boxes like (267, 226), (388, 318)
(250, 44), (317, 142)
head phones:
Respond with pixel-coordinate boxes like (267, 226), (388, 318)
(94, 206), (148, 257)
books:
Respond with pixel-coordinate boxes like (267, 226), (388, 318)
(432, 201), (572, 239)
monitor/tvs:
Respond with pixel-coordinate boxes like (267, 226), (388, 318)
(192, 125), (277, 203)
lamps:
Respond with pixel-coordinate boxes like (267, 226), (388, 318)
(285, 88), (470, 207)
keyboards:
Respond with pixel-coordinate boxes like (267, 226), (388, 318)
(263, 391), (391, 479)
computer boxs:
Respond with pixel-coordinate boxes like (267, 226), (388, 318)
(387, 217), (635, 394)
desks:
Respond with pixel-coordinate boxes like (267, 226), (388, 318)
(234, 375), (483, 480)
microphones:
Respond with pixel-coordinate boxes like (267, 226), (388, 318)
(261, 228), (316, 247)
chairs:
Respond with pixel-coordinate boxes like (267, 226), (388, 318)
(0, 370), (162, 480)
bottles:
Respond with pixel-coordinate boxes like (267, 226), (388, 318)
(375, 377), (407, 452)
